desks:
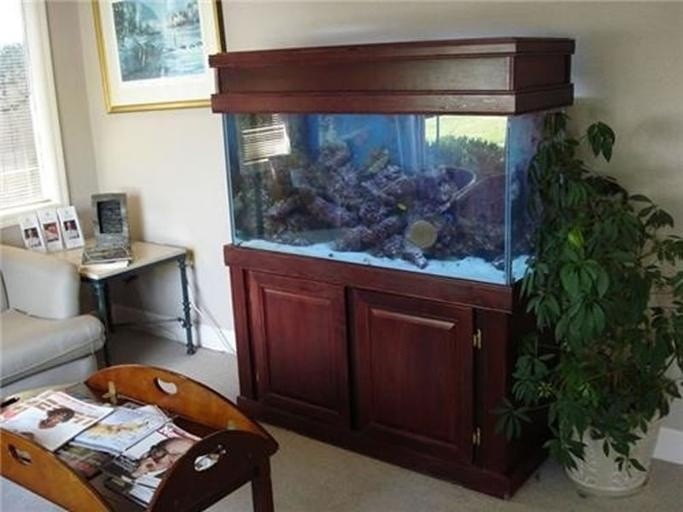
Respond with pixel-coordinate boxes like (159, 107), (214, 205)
(51, 236), (195, 367)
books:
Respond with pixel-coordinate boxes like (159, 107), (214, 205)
(16, 212), (46, 254)
(0, 381), (226, 510)
(56, 204), (83, 250)
(37, 208), (63, 252)
(79, 243), (133, 272)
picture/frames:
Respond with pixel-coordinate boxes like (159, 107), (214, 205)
(92, 0), (226, 114)
(91, 192), (130, 250)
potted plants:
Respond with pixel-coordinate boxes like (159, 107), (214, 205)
(492, 113), (683, 497)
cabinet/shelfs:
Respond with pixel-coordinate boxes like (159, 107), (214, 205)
(223, 243), (550, 499)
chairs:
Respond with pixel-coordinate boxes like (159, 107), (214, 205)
(0, 243), (106, 399)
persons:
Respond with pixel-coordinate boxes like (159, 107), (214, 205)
(45, 223), (58, 241)
(25, 229), (38, 247)
(64, 221), (77, 241)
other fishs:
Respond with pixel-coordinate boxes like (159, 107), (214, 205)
(397, 203), (407, 210)
(406, 220), (443, 248)
